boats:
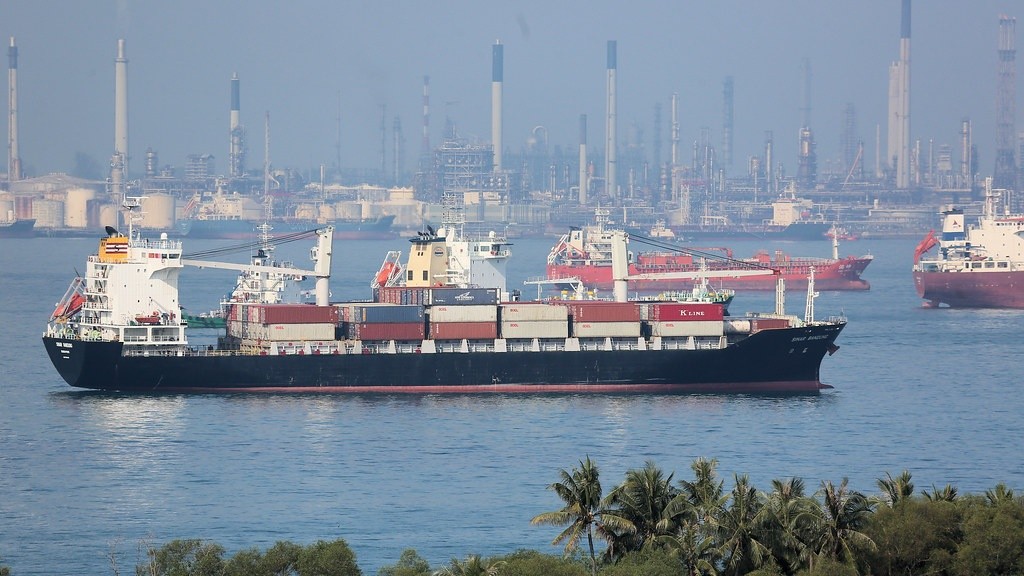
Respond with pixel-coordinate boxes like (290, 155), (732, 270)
(911, 178), (1024, 311)
(440, 193), (539, 239)
(164, 199), (396, 240)
(568, 178), (875, 242)
(545, 201), (875, 293)
(43, 193), (847, 401)
(1, 216), (39, 236)
